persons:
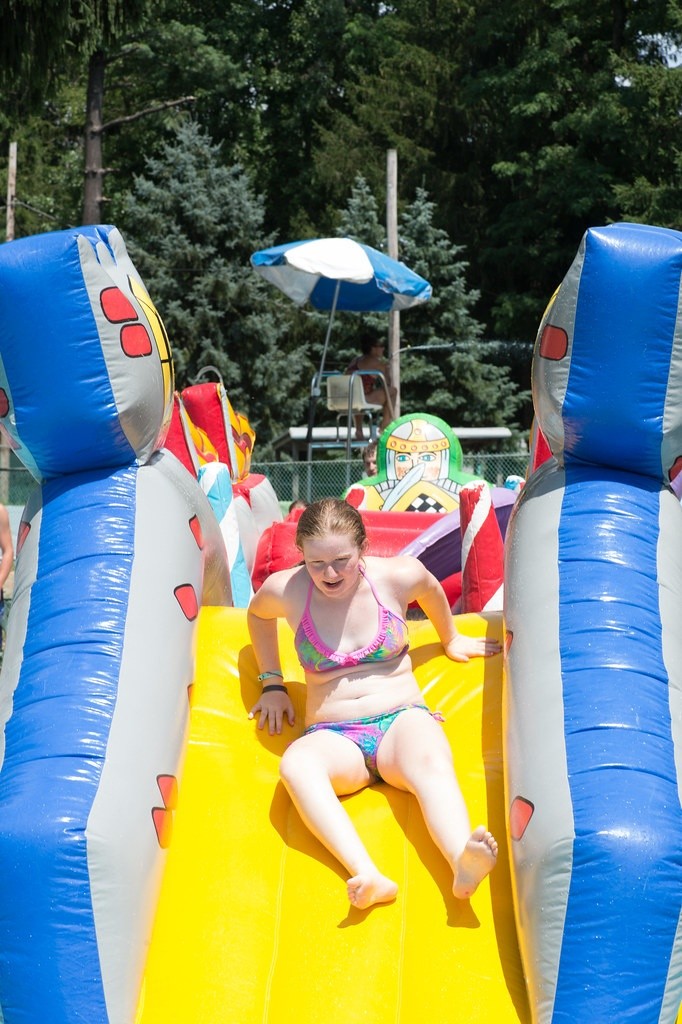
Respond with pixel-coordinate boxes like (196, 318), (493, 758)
(345, 339), (397, 439)
(0, 504), (14, 661)
(362, 441), (378, 477)
(289, 500), (310, 512)
(247, 498), (503, 910)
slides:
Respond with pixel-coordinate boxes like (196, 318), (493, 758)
(0, 601), (682, 1024)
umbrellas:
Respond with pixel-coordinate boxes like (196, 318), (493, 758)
(250, 239), (432, 439)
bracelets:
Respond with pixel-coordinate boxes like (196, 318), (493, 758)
(263, 685), (287, 694)
(257, 669), (284, 681)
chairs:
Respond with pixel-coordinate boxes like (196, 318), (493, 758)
(326, 374), (385, 441)
